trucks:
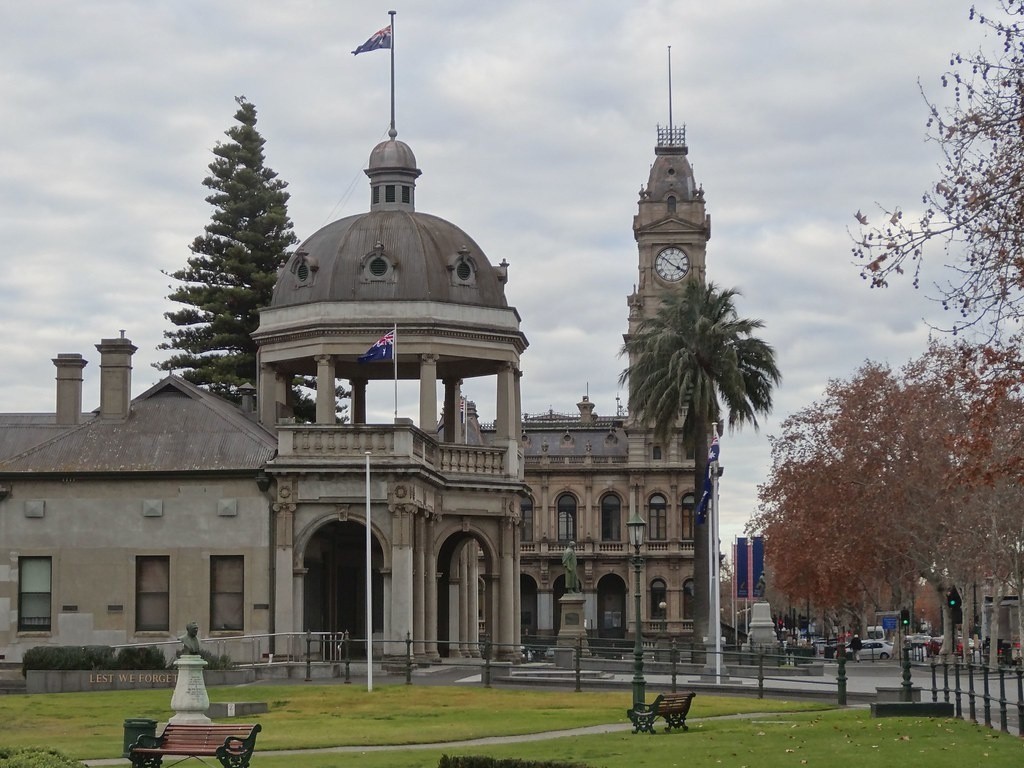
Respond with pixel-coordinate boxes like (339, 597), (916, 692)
(981, 594), (1024, 665)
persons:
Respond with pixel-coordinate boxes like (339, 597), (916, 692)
(561, 541), (579, 593)
(175, 623), (199, 658)
(851, 634), (862, 663)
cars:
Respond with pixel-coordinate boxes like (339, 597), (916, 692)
(834, 639), (894, 661)
(779, 637), (838, 654)
(902, 634), (974, 662)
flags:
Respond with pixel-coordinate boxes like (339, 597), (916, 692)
(357, 331), (395, 362)
(460, 399), (466, 423)
(695, 480), (711, 524)
(351, 25), (391, 56)
(705, 433), (719, 489)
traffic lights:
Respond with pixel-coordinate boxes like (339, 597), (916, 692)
(847, 626), (851, 636)
(901, 610), (910, 626)
(948, 591), (959, 606)
(778, 618), (783, 630)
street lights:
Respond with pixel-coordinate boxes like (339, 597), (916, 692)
(626, 511), (648, 734)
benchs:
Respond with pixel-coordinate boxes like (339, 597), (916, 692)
(127, 722), (262, 768)
(627, 691), (697, 734)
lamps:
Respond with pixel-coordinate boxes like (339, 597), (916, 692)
(0, 488), (10, 503)
(255, 477), (271, 493)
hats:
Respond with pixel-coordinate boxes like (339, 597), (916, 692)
(854, 634), (858, 638)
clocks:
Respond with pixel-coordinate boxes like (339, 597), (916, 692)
(655, 247), (689, 281)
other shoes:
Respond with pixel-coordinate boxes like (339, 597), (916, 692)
(857, 660), (859, 663)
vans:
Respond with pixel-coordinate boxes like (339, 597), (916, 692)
(866, 625), (884, 643)
